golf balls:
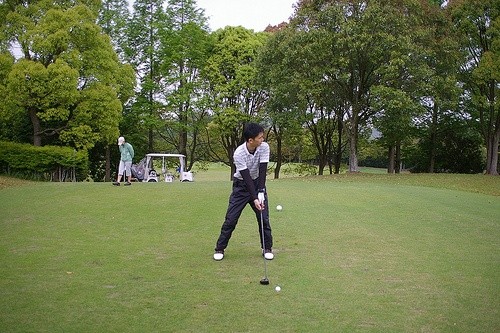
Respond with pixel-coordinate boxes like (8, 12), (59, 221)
(277, 206), (282, 210)
(275, 286), (281, 291)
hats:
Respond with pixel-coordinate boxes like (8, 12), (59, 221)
(118, 137), (125, 145)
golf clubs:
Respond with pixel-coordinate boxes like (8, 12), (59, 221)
(260, 204), (269, 285)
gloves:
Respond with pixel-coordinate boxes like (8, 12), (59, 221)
(258, 193), (265, 206)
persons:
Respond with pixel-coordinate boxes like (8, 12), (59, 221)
(212, 123), (276, 260)
(113, 136), (135, 187)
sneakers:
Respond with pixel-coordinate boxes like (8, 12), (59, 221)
(124, 182), (131, 185)
(112, 182), (120, 186)
(214, 253), (224, 260)
(263, 250), (274, 260)
